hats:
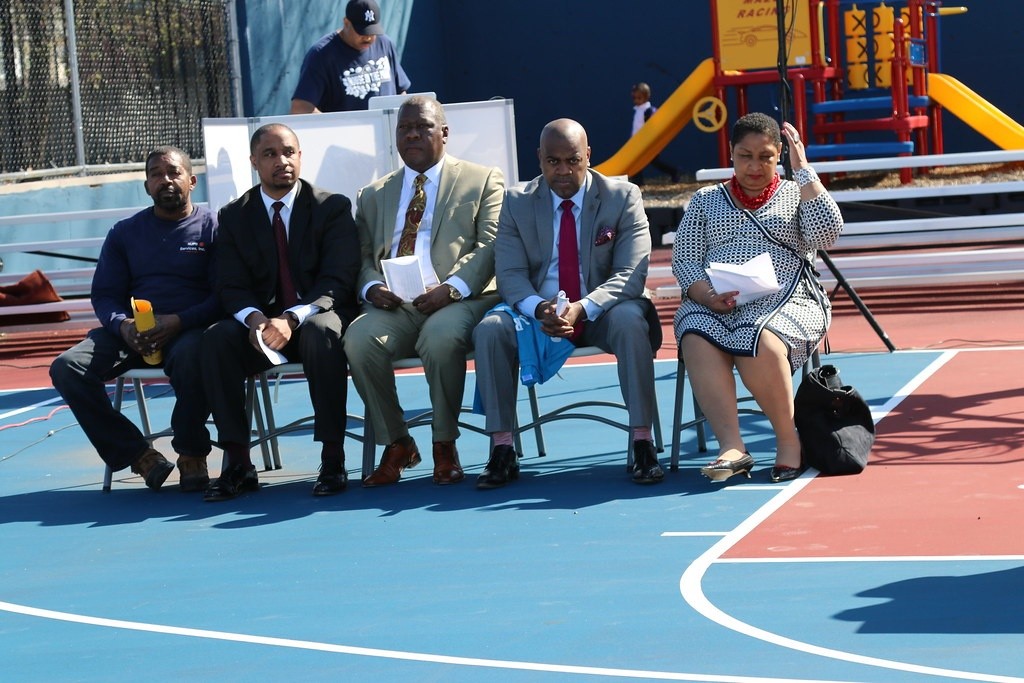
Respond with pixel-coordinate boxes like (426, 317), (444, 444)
(346, 0), (384, 35)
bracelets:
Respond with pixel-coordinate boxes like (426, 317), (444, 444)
(792, 166), (821, 188)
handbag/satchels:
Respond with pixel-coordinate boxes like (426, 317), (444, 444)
(794, 365), (875, 476)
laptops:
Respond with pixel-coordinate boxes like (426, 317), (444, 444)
(368, 92), (437, 110)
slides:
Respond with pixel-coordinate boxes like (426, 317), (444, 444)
(926, 70), (1023, 149)
(590, 56), (717, 179)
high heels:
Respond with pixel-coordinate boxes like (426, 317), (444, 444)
(701, 455), (755, 479)
(770, 443), (810, 482)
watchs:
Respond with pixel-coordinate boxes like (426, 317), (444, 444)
(286, 311), (300, 327)
(448, 285), (462, 302)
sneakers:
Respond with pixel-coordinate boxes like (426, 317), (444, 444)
(137, 448), (175, 489)
(177, 454), (210, 491)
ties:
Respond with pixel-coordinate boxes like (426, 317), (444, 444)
(396, 172), (428, 256)
(558, 200), (584, 341)
(271, 202), (297, 316)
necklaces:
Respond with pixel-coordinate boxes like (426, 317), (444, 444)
(730, 172), (780, 209)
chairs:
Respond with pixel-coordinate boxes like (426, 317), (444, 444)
(102, 342), (823, 499)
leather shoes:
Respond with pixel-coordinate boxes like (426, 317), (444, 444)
(312, 463), (348, 494)
(361, 437), (421, 487)
(433, 440), (466, 484)
(201, 464), (259, 502)
(632, 439), (664, 482)
(476, 445), (520, 487)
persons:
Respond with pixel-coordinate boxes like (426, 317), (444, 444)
(289, 0), (411, 114)
(628, 82), (684, 186)
(671, 112), (843, 483)
(342, 95), (506, 487)
(49, 146), (220, 494)
(470, 118), (665, 488)
(191, 122), (361, 502)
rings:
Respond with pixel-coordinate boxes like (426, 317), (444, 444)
(383, 305), (388, 309)
(794, 140), (800, 144)
